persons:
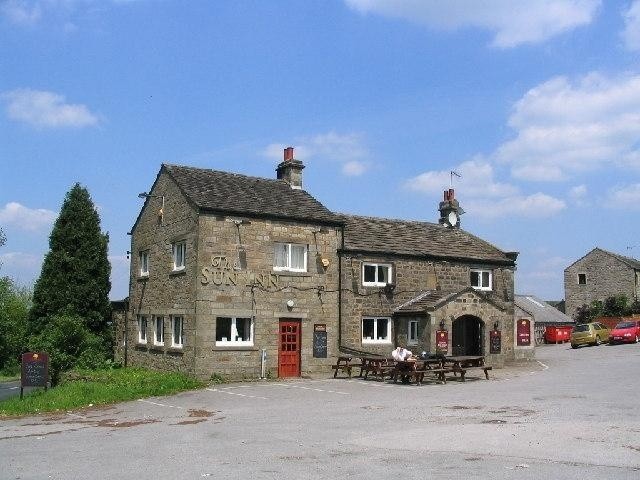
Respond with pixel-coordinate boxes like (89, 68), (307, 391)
(391, 344), (413, 385)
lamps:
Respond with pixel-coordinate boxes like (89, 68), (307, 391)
(234, 219), (251, 228)
(440, 319), (445, 328)
(313, 228), (329, 236)
(138, 192), (164, 199)
(494, 321), (498, 327)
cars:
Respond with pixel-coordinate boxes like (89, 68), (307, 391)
(570, 321), (640, 349)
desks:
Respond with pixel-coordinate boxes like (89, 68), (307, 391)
(332, 355), (490, 386)
